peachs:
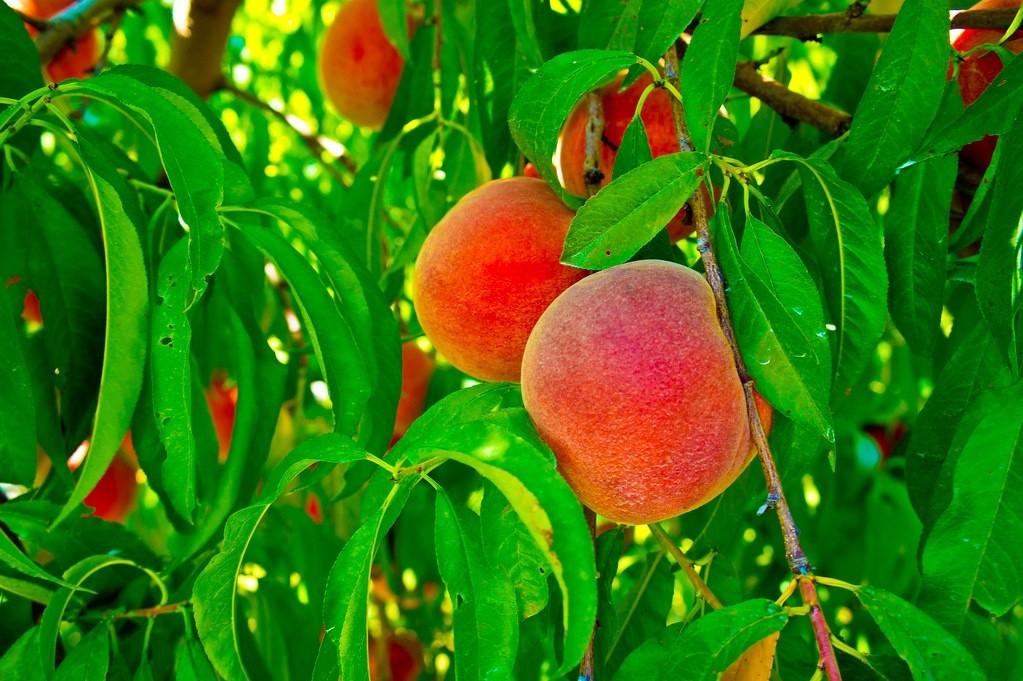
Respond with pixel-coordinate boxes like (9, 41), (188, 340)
(1, 1), (1023, 680)
